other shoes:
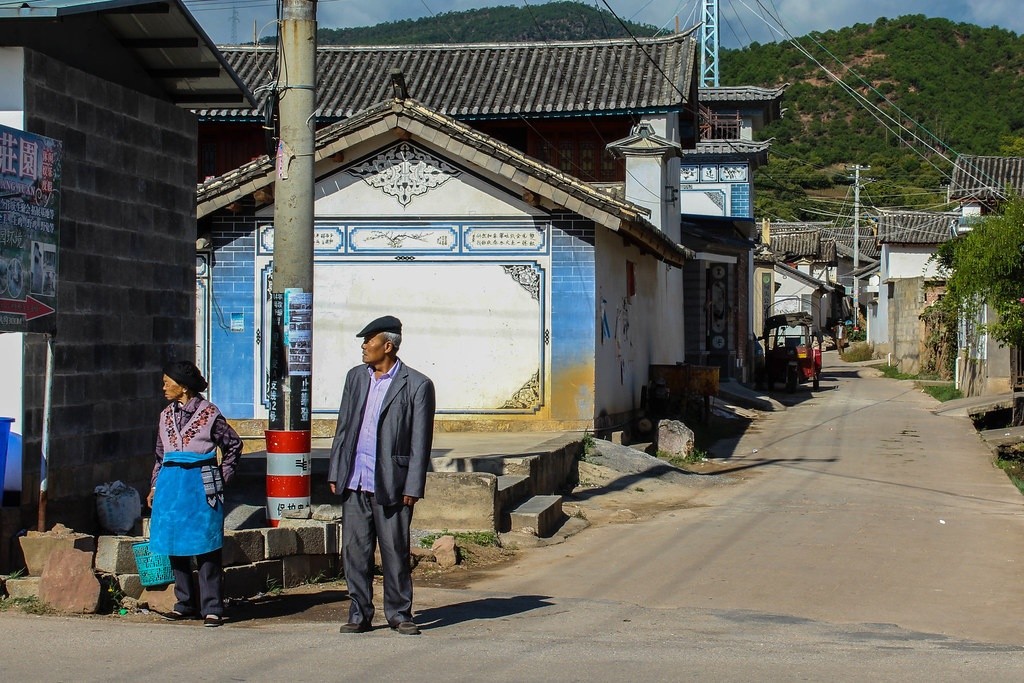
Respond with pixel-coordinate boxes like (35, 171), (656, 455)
(204, 615), (223, 627)
(163, 611), (200, 621)
(339, 622), (372, 633)
(393, 621), (419, 635)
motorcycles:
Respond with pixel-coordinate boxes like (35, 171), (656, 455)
(761, 314), (823, 391)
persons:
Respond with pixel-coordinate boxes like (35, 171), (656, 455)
(328, 315), (435, 635)
(831, 319), (847, 355)
(147, 361), (244, 626)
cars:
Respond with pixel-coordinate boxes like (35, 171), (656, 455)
(752, 332), (765, 367)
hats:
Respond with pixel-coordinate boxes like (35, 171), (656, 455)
(355, 315), (402, 338)
